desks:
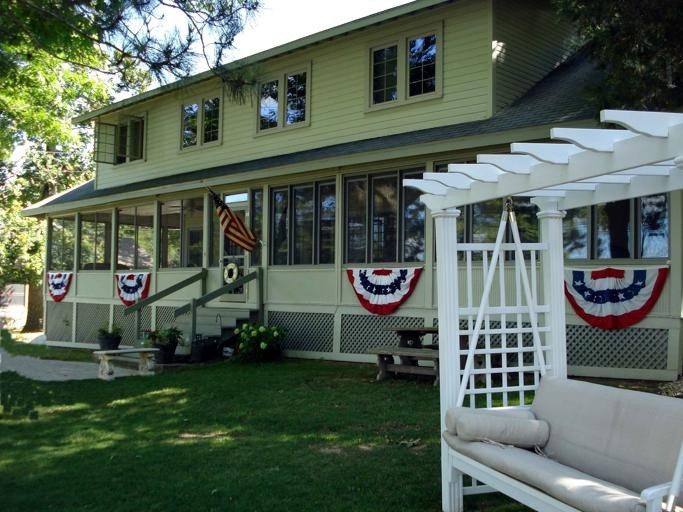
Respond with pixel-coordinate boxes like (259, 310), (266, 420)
(382, 327), (438, 367)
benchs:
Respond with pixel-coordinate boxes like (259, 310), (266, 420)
(94, 348), (160, 380)
(368, 345), (440, 387)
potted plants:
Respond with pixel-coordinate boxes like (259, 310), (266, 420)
(148, 326), (184, 363)
(98, 326), (123, 355)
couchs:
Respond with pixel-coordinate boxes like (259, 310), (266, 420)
(443, 374), (683, 512)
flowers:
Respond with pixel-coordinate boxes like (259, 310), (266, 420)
(234, 323), (287, 350)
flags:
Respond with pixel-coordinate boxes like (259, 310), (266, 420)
(205, 186), (258, 254)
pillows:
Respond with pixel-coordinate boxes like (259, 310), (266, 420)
(456, 414), (550, 448)
(445, 408), (536, 435)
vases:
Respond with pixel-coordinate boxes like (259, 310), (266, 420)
(244, 348), (261, 363)
